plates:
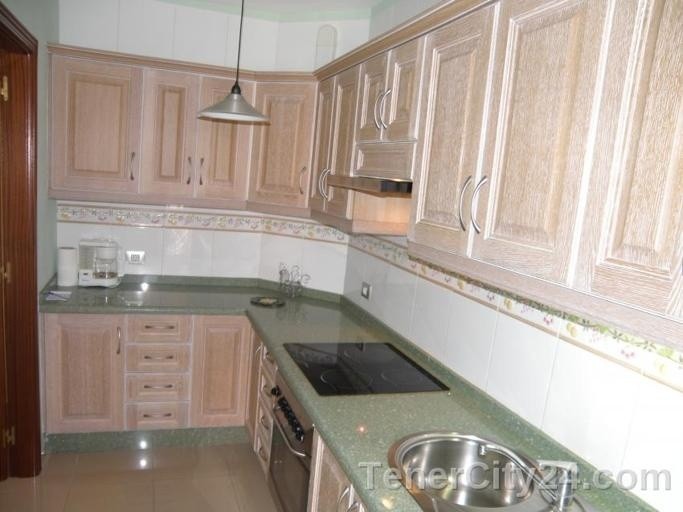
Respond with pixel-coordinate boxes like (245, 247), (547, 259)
(251, 295), (285, 306)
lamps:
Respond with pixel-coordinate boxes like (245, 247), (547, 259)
(198, 0), (269, 124)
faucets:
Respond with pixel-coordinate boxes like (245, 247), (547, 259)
(477, 442), (581, 510)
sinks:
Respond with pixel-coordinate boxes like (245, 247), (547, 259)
(389, 431), (534, 507)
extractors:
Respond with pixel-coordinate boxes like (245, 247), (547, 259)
(326, 172), (412, 194)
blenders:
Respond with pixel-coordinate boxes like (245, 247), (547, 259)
(77, 239), (119, 289)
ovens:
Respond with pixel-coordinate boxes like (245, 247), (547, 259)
(267, 370), (314, 511)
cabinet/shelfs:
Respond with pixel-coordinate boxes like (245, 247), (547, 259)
(249, 68), (316, 219)
(39, 312), (278, 476)
(142, 56), (257, 212)
(309, 0), (683, 353)
(47, 43), (141, 205)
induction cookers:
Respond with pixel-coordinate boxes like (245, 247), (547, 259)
(283, 342), (451, 397)
